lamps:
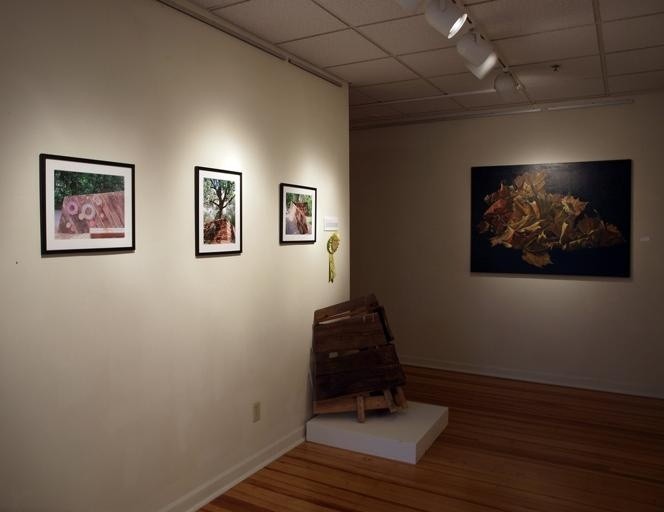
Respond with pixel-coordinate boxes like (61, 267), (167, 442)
(422, 0), (494, 80)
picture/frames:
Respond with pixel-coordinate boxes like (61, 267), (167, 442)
(193, 164), (245, 258)
(37, 152), (138, 258)
(279, 181), (318, 246)
